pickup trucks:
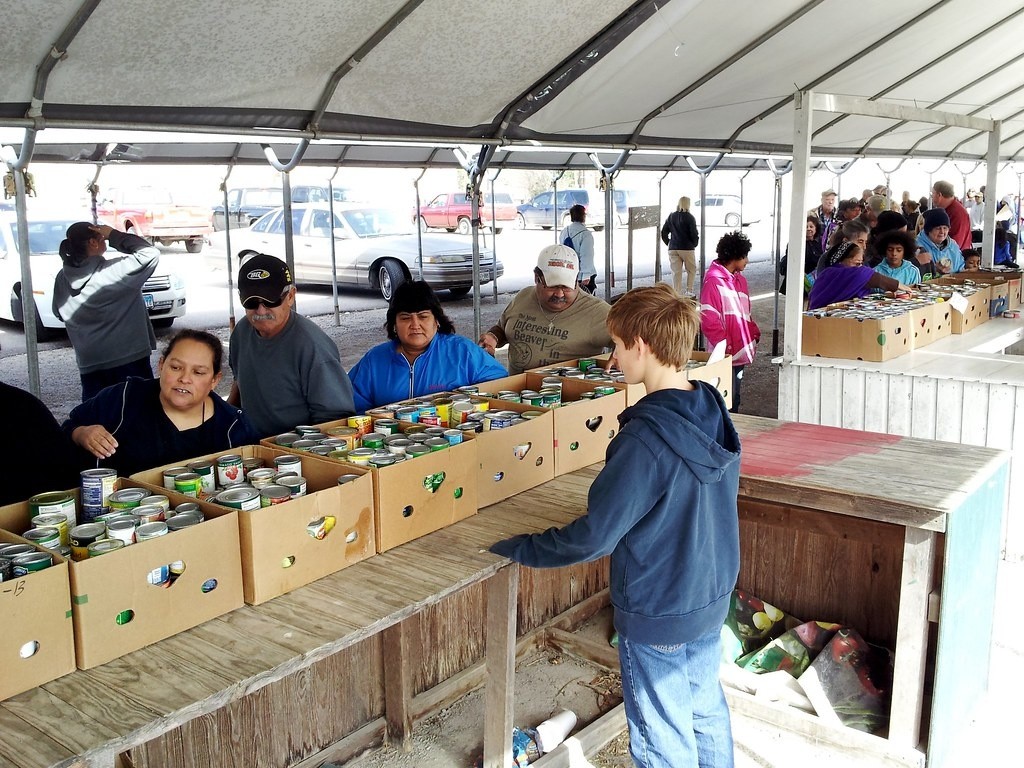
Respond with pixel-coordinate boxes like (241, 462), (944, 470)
(412, 191), (517, 235)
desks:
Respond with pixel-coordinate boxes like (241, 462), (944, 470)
(1, 411), (1008, 768)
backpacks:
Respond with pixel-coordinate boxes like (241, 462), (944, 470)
(563, 226), (588, 252)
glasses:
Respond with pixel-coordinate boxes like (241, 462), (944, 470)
(536, 273), (578, 292)
(239, 290), (290, 309)
(975, 196), (982, 198)
(875, 192), (887, 197)
(863, 200), (868, 206)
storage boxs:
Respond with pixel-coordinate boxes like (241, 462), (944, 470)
(0, 351), (741, 703)
(801, 268), (1024, 363)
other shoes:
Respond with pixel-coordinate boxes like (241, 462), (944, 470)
(685, 291), (696, 298)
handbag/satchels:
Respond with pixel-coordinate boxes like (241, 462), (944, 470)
(778, 255), (787, 275)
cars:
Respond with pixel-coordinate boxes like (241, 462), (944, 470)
(0, 210), (187, 344)
(84, 184), (214, 254)
(222, 201), (504, 302)
(688, 194), (763, 228)
(213, 187), (347, 233)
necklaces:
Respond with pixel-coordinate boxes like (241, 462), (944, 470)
(536, 292), (558, 332)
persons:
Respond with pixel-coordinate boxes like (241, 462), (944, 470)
(489, 282), (741, 768)
(347, 274), (509, 415)
(779, 180), (981, 311)
(60, 328), (256, 478)
(52, 222), (161, 402)
(662, 197), (699, 293)
(225, 252), (357, 444)
(700, 228), (761, 413)
(478, 244), (616, 376)
(965, 186), (1024, 269)
(559, 203), (597, 296)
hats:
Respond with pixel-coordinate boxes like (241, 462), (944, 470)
(238, 253), (293, 302)
(967, 188), (976, 197)
(537, 243), (579, 291)
(845, 198), (865, 210)
(822, 189), (837, 197)
(876, 210), (908, 233)
(1002, 196), (1011, 206)
(865, 195), (891, 218)
(922, 207), (950, 231)
(974, 192), (984, 198)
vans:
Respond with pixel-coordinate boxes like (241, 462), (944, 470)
(600, 188), (634, 226)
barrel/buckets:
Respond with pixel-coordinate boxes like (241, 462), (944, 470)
(1, 345), (722, 623)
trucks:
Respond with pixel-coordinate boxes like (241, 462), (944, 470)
(514, 189), (618, 232)
